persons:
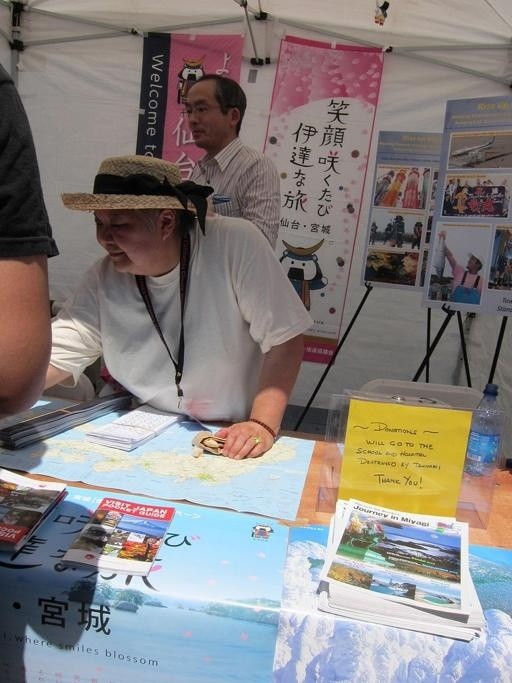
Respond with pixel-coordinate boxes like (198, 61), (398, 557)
(371, 212), (422, 249)
(430, 230), (483, 301)
(0, 63), (59, 420)
(43, 155), (314, 461)
(493, 251), (508, 290)
(187, 75), (281, 254)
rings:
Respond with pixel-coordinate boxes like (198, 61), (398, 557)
(252, 435), (261, 445)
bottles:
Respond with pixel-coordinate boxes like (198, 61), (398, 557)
(459, 382), (505, 515)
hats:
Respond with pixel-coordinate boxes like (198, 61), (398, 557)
(394, 216), (404, 221)
(468, 252), (484, 265)
(415, 222), (422, 227)
(60, 156), (216, 237)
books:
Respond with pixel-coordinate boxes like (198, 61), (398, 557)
(61, 496), (176, 578)
(1, 469), (68, 553)
(1, 390), (134, 451)
(317, 498), (488, 644)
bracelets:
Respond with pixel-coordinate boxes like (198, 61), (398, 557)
(248, 417), (276, 440)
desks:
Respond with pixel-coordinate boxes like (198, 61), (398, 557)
(0, 430), (512, 682)
